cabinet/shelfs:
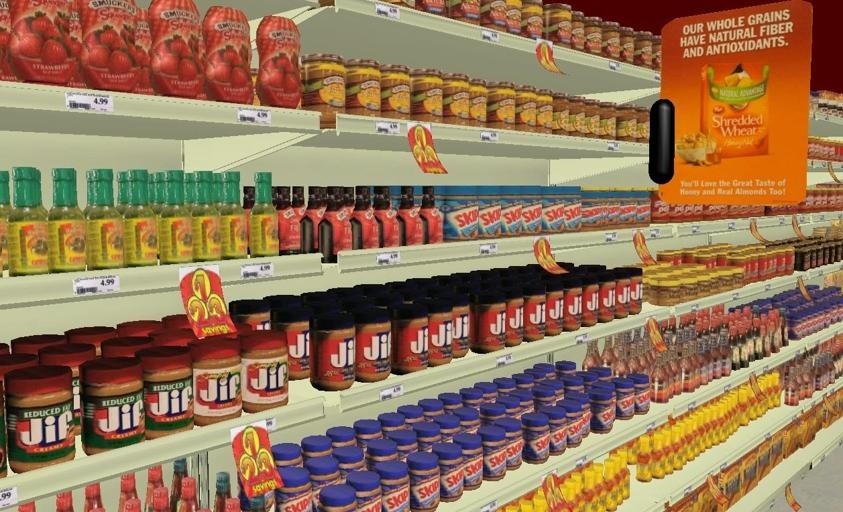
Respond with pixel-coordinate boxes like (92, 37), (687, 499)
(0, 1), (842, 511)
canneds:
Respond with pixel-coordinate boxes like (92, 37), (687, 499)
(249, 0), (662, 143)
(808, 138), (843, 162)
(0, 182), (843, 512)
